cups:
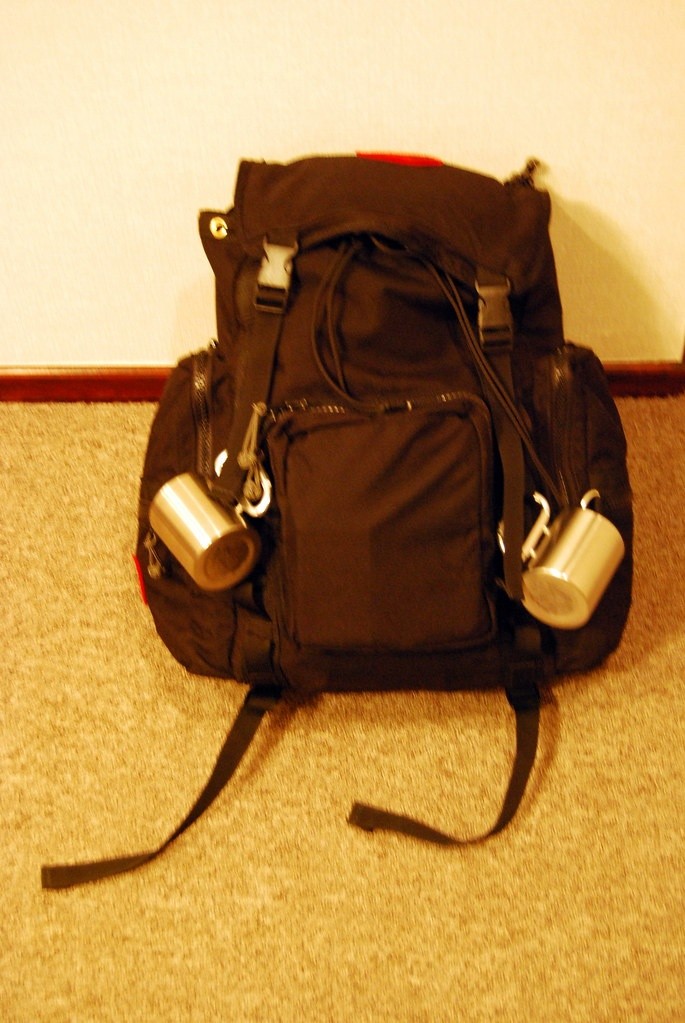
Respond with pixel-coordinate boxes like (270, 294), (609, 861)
(148, 474), (261, 589)
(516, 490), (626, 630)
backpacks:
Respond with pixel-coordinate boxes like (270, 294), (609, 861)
(41, 148), (633, 894)
